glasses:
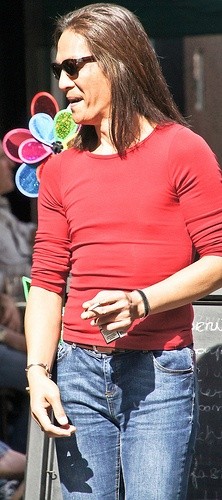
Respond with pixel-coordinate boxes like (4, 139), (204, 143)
(52, 54), (101, 81)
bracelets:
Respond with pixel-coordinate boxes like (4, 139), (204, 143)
(25, 362), (53, 378)
(133, 286), (150, 318)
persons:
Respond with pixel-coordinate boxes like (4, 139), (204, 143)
(0, 141), (37, 499)
(24, 3), (222, 500)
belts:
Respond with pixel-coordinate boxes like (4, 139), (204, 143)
(63, 340), (125, 355)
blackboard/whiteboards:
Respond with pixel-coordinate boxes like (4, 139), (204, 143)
(23, 288), (222, 500)
